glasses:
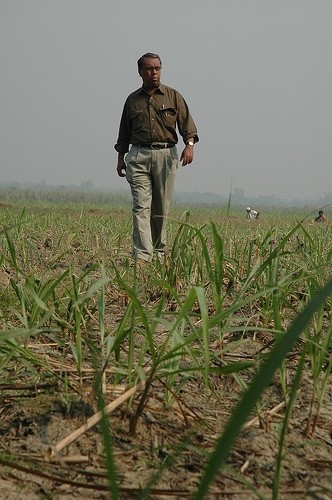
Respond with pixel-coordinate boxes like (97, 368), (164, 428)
(140, 66), (162, 71)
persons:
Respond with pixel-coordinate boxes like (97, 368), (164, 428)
(112, 52), (200, 262)
(315, 210), (328, 223)
(245, 206), (259, 219)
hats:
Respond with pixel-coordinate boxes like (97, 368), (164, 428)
(246, 207), (250, 210)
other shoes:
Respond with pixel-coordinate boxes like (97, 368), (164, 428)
(136, 258), (145, 267)
(153, 252), (161, 257)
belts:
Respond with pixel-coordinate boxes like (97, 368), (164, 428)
(133, 143), (175, 150)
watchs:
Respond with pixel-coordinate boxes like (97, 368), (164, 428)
(186, 141), (194, 146)
